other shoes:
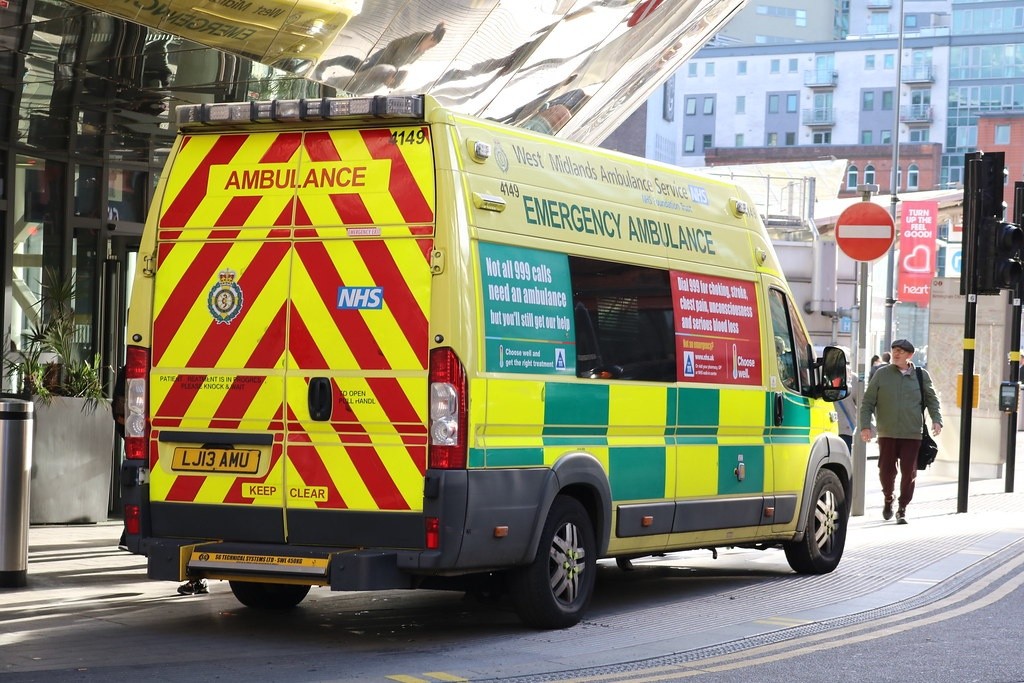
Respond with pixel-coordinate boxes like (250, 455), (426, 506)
(315, 68), (324, 81)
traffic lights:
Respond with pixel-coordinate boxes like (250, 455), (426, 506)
(987, 221), (1023, 290)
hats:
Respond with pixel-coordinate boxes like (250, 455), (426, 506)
(891, 339), (914, 353)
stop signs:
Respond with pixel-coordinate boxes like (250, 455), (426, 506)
(835, 201), (895, 261)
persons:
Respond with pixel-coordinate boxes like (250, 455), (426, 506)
(315, 23), (445, 95)
(111, 364), (127, 552)
(871, 355), (880, 366)
(1019, 354), (1024, 390)
(832, 366), (857, 451)
(869, 351), (891, 384)
(859, 340), (944, 524)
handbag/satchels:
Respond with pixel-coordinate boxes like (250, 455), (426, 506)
(917, 431), (938, 470)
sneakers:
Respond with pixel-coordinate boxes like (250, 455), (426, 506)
(178, 578), (209, 594)
(895, 510), (907, 524)
(883, 495), (895, 520)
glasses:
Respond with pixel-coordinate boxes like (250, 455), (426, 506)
(892, 349), (907, 354)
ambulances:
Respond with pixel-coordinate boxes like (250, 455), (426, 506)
(112, 94), (856, 631)
